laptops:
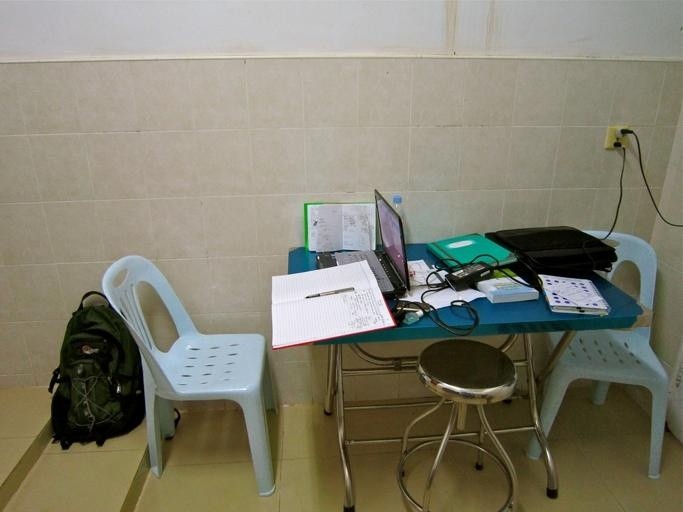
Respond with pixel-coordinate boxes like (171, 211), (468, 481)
(315, 189), (410, 301)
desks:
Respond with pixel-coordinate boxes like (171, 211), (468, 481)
(287, 243), (653, 512)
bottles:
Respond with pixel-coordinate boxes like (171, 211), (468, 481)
(391, 195), (407, 254)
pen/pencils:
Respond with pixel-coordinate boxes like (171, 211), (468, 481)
(305, 287), (354, 298)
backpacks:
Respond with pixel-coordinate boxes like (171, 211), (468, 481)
(46, 290), (145, 449)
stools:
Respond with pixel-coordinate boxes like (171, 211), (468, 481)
(398, 337), (516, 512)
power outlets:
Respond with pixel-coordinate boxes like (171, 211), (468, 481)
(605, 124), (630, 149)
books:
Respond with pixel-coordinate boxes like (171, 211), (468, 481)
(270, 259), (397, 349)
(472, 267), (540, 304)
(537, 272), (613, 317)
(303, 200), (383, 252)
(426, 233), (519, 272)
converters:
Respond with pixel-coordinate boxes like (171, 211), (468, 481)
(446, 261), (493, 292)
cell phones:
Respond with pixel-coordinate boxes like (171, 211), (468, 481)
(396, 301), (430, 312)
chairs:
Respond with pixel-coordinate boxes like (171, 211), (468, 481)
(103, 252), (276, 496)
(525, 227), (669, 480)
